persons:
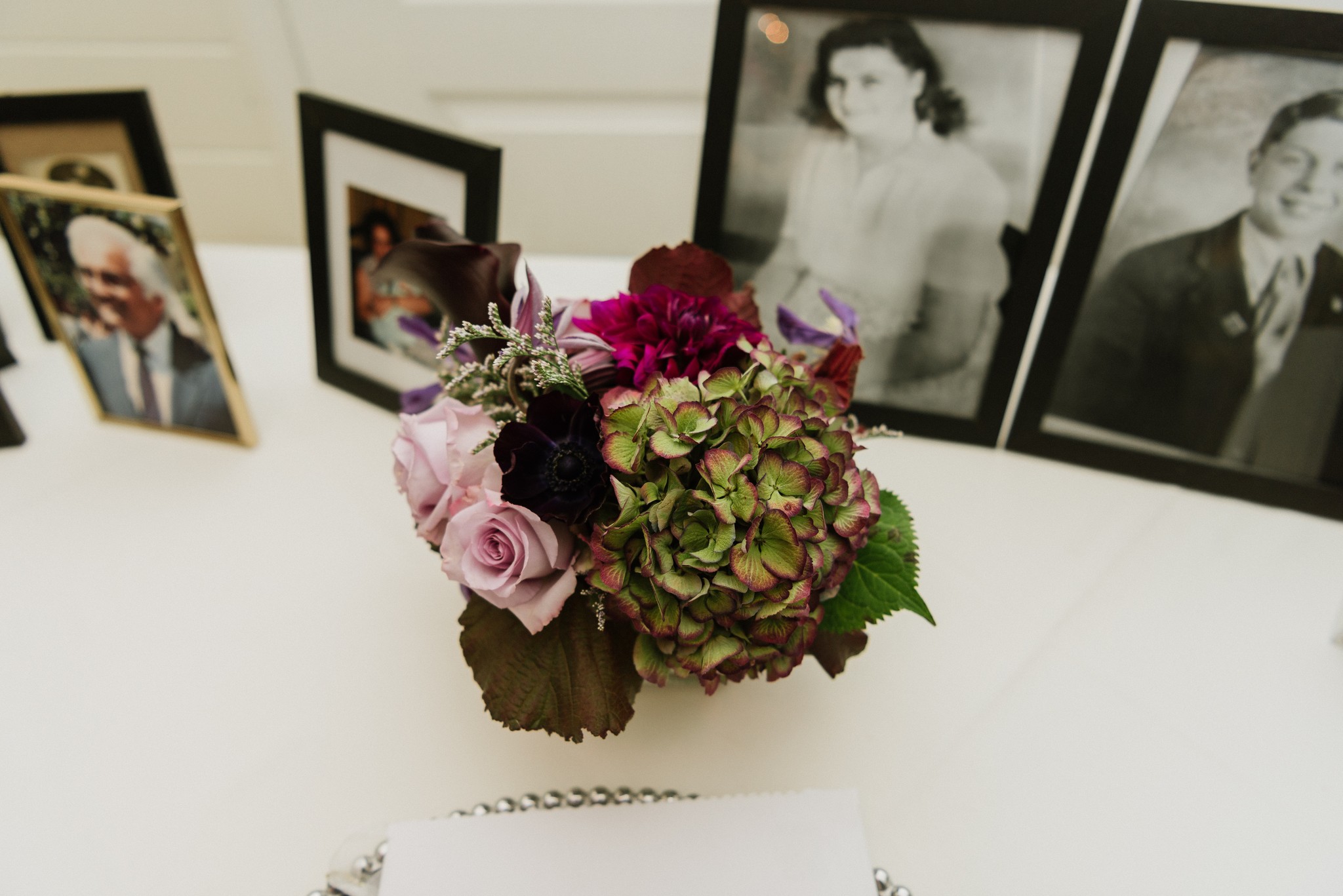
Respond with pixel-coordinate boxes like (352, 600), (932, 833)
(357, 205), (433, 352)
(747, 12), (1011, 418)
(64, 216), (234, 434)
(1051, 89), (1342, 490)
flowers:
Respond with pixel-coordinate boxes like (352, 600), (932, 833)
(367, 218), (937, 745)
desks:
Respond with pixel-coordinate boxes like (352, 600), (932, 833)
(0, 240), (1342, 894)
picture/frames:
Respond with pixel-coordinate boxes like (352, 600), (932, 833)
(0, 91), (178, 343)
(0, 172), (262, 450)
(298, 92), (505, 416)
(691, 0), (1128, 449)
(1003, 1), (1342, 524)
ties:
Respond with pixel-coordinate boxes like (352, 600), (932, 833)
(1250, 256), (1307, 387)
(133, 343), (161, 422)
